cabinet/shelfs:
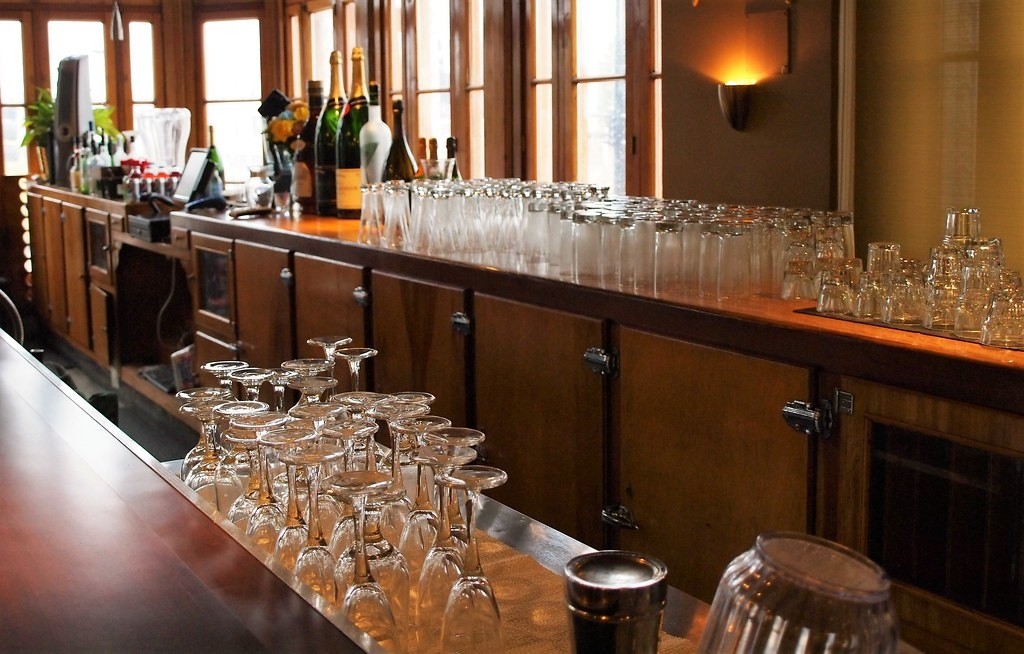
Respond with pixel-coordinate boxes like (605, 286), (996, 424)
(26, 177), (1020, 654)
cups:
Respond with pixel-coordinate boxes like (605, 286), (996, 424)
(273, 191), (291, 211)
(697, 532), (900, 654)
(359, 176), (1024, 349)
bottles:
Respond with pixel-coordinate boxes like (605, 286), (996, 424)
(208, 123), (225, 196)
(359, 81), (393, 185)
(69, 119), (182, 201)
(314, 49), (348, 216)
(336, 46), (370, 218)
(245, 171), (272, 208)
(425, 139), (443, 180)
(441, 135), (461, 181)
(290, 80), (326, 213)
(378, 94), (418, 227)
(563, 550), (668, 653)
(414, 138), (428, 180)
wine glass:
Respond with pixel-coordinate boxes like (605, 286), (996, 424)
(177, 336), (509, 652)
